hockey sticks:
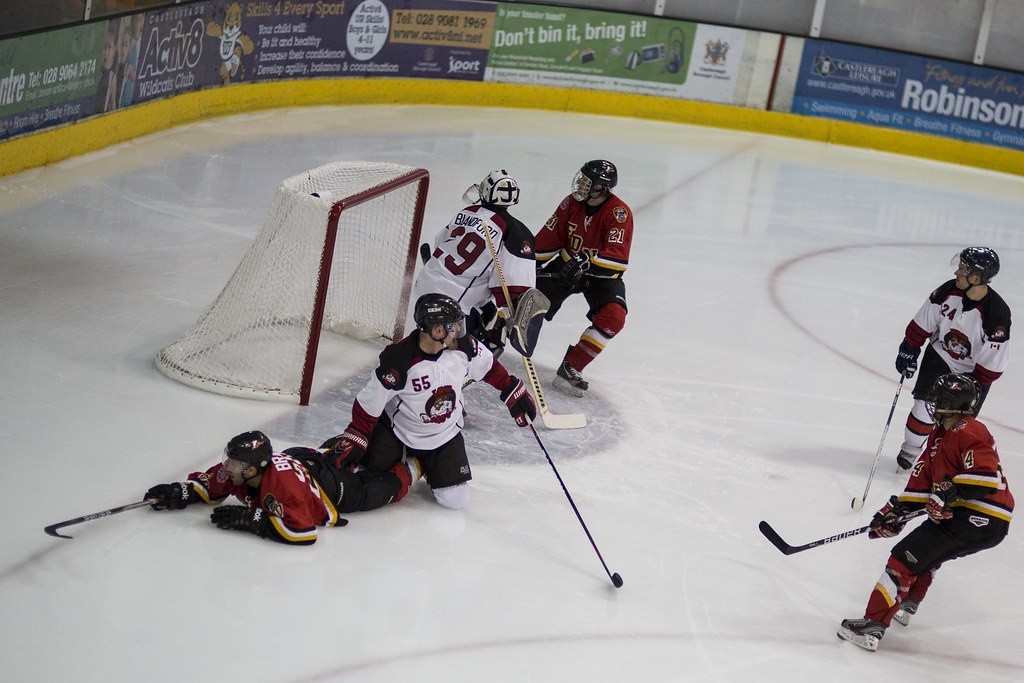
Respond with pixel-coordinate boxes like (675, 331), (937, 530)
(44, 497), (160, 539)
(851, 367), (908, 510)
(523, 412), (619, 587)
(480, 221), (587, 429)
(758, 507), (930, 556)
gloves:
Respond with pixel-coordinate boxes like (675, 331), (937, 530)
(499, 375), (537, 428)
(143, 482), (194, 510)
(561, 251), (589, 281)
(324, 430), (368, 472)
(868, 495), (907, 539)
(209, 505), (265, 536)
(895, 349), (917, 378)
(927, 482), (955, 524)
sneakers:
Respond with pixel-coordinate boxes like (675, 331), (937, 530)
(836, 616), (887, 653)
(552, 362), (589, 398)
(892, 597), (919, 626)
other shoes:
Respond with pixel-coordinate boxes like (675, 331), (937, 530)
(895, 449), (916, 474)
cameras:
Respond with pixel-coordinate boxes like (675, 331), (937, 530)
(642, 44), (666, 63)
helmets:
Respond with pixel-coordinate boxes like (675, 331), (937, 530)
(926, 372), (977, 422)
(414, 293), (467, 338)
(958, 247), (1001, 282)
(572, 159), (618, 201)
(224, 430), (273, 475)
(479, 168), (520, 205)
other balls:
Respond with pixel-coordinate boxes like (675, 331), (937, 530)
(612, 573), (623, 588)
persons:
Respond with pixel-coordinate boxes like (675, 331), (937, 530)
(893, 245), (1012, 475)
(409, 169), (535, 362)
(144, 429), (424, 549)
(838, 372), (1014, 653)
(327, 293), (538, 511)
(96, 15), (144, 114)
(527, 159), (633, 396)
